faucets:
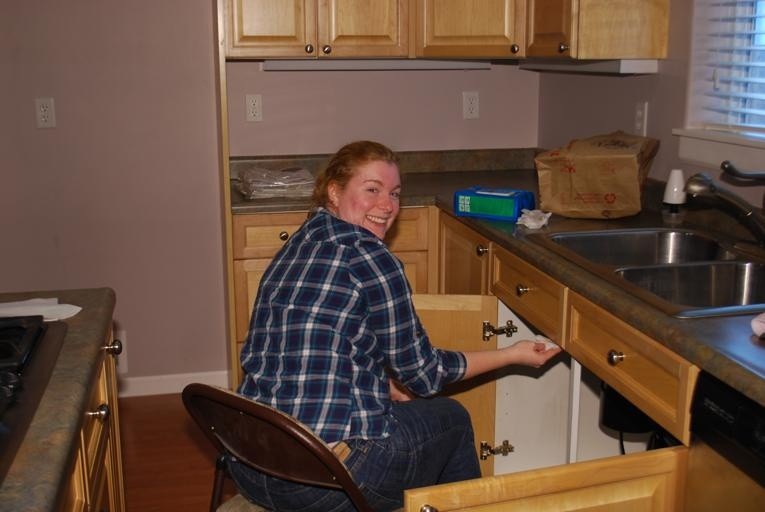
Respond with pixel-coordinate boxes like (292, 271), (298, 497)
(683, 161), (764, 247)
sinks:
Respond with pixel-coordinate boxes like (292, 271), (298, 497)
(613, 259), (765, 319)
(546, 226), (744, 272)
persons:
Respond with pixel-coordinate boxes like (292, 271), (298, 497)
(228, 140), (564, 510)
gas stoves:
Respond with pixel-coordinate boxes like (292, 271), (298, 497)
(0, 314), (67, 459)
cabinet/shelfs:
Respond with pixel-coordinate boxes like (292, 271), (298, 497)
(318, 0), (410, 58)
(527, 0), (669, 60)
(60, 321), (126, 511)
(438, 210), (493, 294)
(232, 210), (308, 397)
(375, 207), (430, 295)
(223, 1), (318, 59)
(411, 0), (528, 61)
(395, 246), (698, 512)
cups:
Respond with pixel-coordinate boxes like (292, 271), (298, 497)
(664, 168), (688, 205)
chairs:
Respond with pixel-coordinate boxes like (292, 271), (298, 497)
(183, 383), (367, 512)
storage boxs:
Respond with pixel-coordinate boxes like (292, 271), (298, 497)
(533, 130), (660, 217)
